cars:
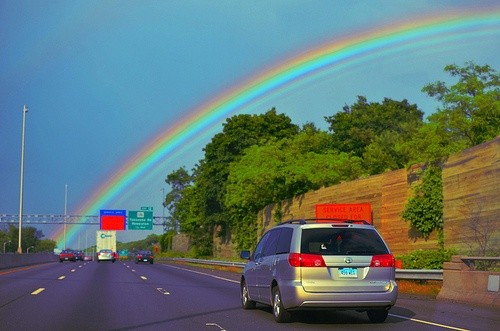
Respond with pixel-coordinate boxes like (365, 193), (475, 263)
(74, 250), (85, 261)
(83, 252), (92, 261)
(97, 250), (116, 263)
(134, 250), (154, 264)
(59, 249), (77, 263)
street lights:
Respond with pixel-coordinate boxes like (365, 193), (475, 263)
(16, 105), (29, 253)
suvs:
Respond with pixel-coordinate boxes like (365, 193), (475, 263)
(239, 218), (398, 324)
